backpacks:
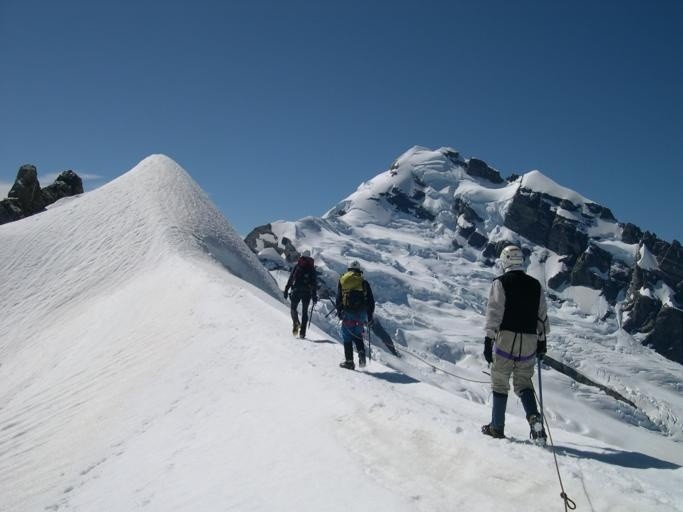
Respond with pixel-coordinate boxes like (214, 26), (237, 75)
(292, 256), (314, 285)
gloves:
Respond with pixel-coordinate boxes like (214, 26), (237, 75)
(536, 339), (547, 360)
(483, 336), (493, 364)
(312, 293), (317, 303)
(284, 289), (288, 299)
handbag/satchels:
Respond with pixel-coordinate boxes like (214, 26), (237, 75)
(340, 270), (369, 313)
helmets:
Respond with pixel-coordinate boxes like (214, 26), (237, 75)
(300, 250), (311, 257)
(347, 259), (360, 269)
(500, 245), (525, 274)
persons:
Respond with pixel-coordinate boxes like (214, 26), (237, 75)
(481, 244), (551, 447)
(336, 260), (375, 370)
(283, 249), (318, 339)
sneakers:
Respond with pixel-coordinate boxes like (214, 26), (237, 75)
(358, 352), (367, 368)
(526, 410), (547, 442)
(482, 422), (505, 438)
(340, 361), (355, 370)
(299, 327), (306, 338)
(293, 318), (300, 335)
